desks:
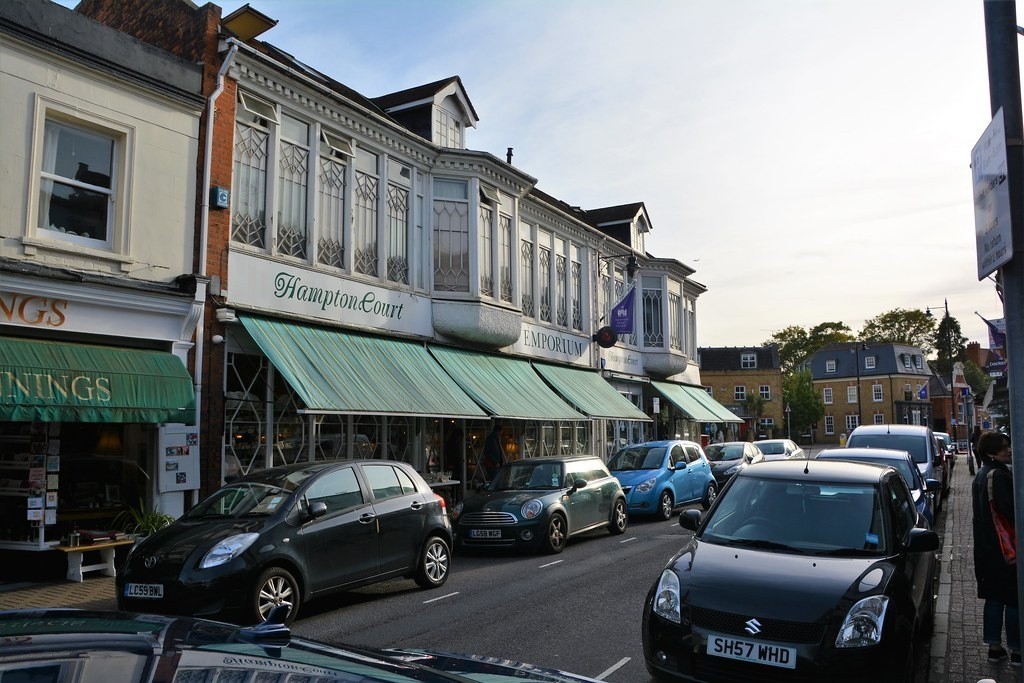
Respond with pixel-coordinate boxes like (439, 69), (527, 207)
(428, 480), (460, 506)
(49, 539), (134, 583)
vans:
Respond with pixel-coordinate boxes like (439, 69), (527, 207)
(274, 433), (372, 465)
(845, 424), (943, 514)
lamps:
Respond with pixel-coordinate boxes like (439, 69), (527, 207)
(95, 423), (124, 455)
(598, 249), (641, 278)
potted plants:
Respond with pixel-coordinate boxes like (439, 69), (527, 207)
(112, 496), (176, 544)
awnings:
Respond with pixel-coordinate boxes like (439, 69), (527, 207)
(650, 380), (745, 423)
(239, 315), (650, 421)
(1, 338), (198, 428)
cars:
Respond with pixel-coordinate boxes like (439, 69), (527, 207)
(114, 458), (454, 627)
(0, 606), (609, 683)
(702, 441), (766, 490)
(641, 457), (941, 683)
(753, 439), (806, 461)
(449, 455), (629, 556)
(795, 448), (941, 530)
(931, 431), (958, 501)
(606, 439), (720, 522)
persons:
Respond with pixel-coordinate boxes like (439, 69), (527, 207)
(485, 425), (504, 471)
(714, 426), (734, 443)
(972, 433), (1024, 667)
(970, 423), (981, 468)
(446, 429), (462, 503)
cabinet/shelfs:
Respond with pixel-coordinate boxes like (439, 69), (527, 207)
(0, 435), (33, 497)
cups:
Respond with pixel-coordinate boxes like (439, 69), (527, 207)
(419, 471), (452, 484)
(68, 531), (80, 547)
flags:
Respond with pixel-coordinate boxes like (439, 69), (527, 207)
(611, 287), (635, 334)
(982, 317), (1006, 347)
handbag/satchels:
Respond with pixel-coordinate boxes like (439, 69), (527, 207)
(992, 512), (1017, 564)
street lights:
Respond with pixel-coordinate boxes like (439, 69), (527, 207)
(851, 342), (870, 427)
(926, 299), (960, 455)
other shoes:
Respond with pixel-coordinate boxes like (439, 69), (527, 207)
(1010, 652), (1023, 666)
(988, 647), (1007, 662)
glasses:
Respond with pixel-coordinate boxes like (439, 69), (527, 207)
(1001, 444), (1011, 450)
